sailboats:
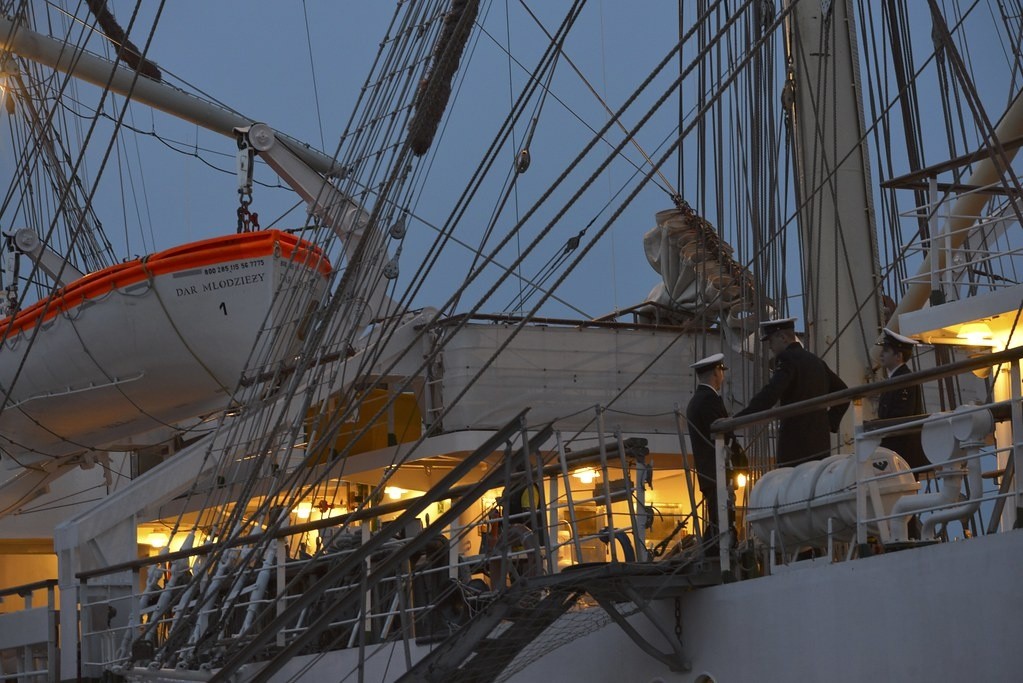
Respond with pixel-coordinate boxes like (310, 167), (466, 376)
(0, 0), (1023, 681)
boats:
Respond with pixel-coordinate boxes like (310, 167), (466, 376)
(0, 229), (334, 461)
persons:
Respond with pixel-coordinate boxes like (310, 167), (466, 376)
(874, 327), (932, 540)
(685, 353), (751, 557)
(479, 508), (503, 553)
(510, 460), (544, 546)
(713, 316), (851, 469)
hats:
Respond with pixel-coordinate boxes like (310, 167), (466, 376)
(758, 317), (798, 341)
(874, 328), (919, 355)
(689, 353), (730, 373)
